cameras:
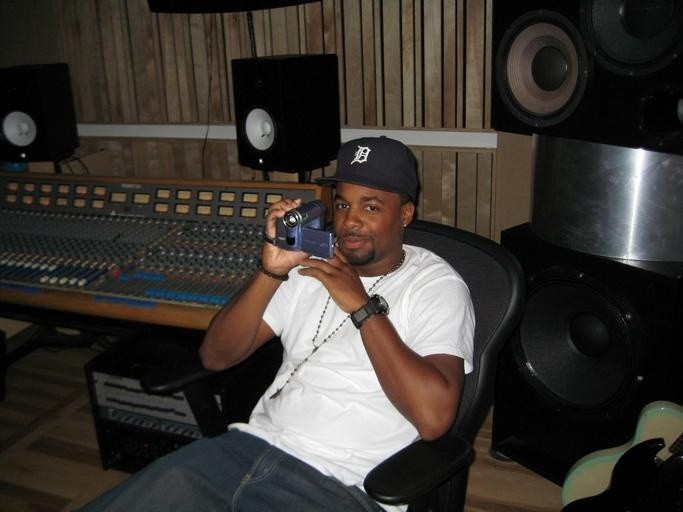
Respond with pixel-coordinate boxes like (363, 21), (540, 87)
(276, 200), (334, 259)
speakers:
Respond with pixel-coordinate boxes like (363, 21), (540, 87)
(492, 221), (683, 487)
(0, 63), (79, 162)
(491, 0), (683, 155)
(231, 54), (340, 173)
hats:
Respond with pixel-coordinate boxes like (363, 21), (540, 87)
(314, 135), (420, 206)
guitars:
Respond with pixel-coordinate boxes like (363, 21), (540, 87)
(562, 402), (682, 511)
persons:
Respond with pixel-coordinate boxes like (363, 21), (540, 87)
(74, 137), (479, 511)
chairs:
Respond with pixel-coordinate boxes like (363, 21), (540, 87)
(139, 216), (528, 512)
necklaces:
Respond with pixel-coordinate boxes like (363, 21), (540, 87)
(268, 248), (405, 399)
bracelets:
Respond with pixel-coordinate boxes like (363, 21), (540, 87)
(257, 261), (291, 282)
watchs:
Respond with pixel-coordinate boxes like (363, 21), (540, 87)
(349, 293), (391, 329)
